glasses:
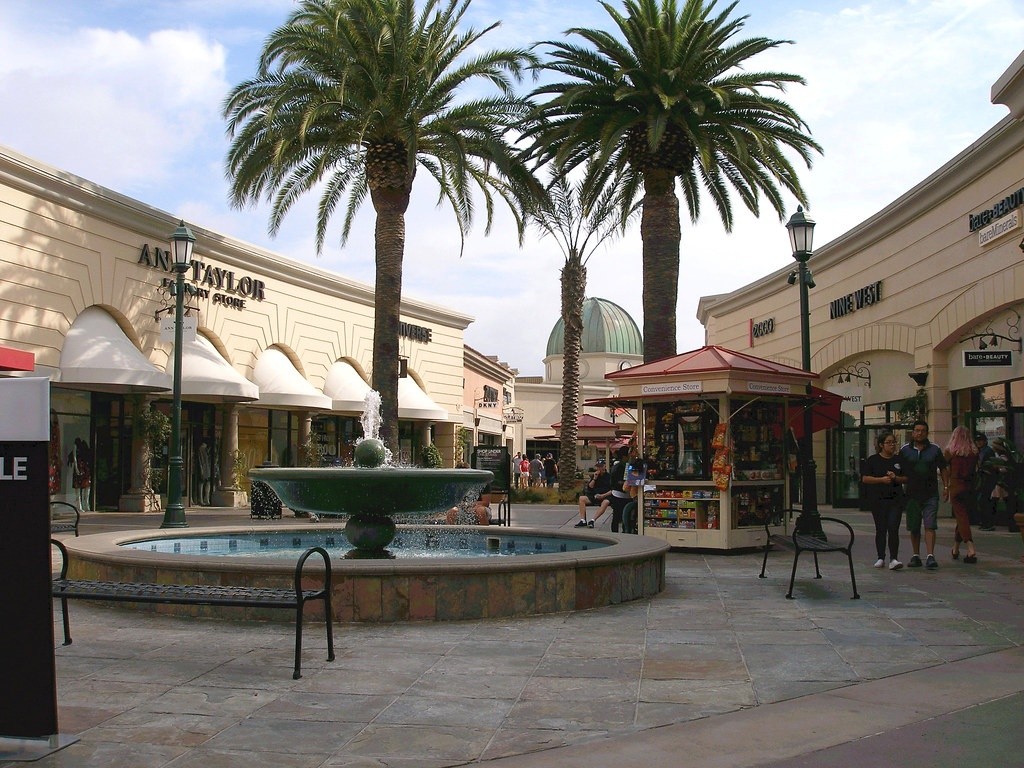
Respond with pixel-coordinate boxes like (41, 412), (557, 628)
(884, 441), (897, 446)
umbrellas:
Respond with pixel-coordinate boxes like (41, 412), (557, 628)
(770, 386), (844, 441)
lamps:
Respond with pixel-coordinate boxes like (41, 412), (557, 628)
(826, 361), (871, 388)
(958, 320), (1022, 350)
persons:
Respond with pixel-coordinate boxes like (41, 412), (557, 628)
(899, 421), (950, 570)
(510, 451), (556, 488)
(947, 424), (978, 563)
(196, 442), (212, 506)
(861, 431), (909, 571)
(975, 431), (998, 531)
(612, 446), (638, 533)
(446, 462), (491, 525)
(67, 437), (92, 513)
(573, 458), (610, 529)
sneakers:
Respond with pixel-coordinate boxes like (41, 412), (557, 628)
(926, 556), (938, 567)
(575, 521), (587, 528)
(908, 556), (923, 567)
(587, 521), (594, 528)
(874, 559), (885, 568)
(888, 560), (903, 570)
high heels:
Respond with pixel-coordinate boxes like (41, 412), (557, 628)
(963, 555), (977, 563)
(952, 548), (960, 559)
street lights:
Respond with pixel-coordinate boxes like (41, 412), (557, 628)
(160, 219), (197, 530)
(785, 204), (827, 541)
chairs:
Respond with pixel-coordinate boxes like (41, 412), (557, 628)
(50, 502), (81, 540)
(582, 479), (614, 524)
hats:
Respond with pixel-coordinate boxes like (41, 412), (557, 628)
(974, 433), (988, 440)
(595, 461), (605, 466)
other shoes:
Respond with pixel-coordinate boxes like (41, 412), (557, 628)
(1008, 525), (1021, 532)
(978, 525), (995, 531)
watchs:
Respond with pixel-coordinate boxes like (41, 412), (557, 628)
(943, 486), (948, 490)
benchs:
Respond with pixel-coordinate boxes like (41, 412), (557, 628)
(50, 540), (335, 681)
(758, 509), (860, 600)
